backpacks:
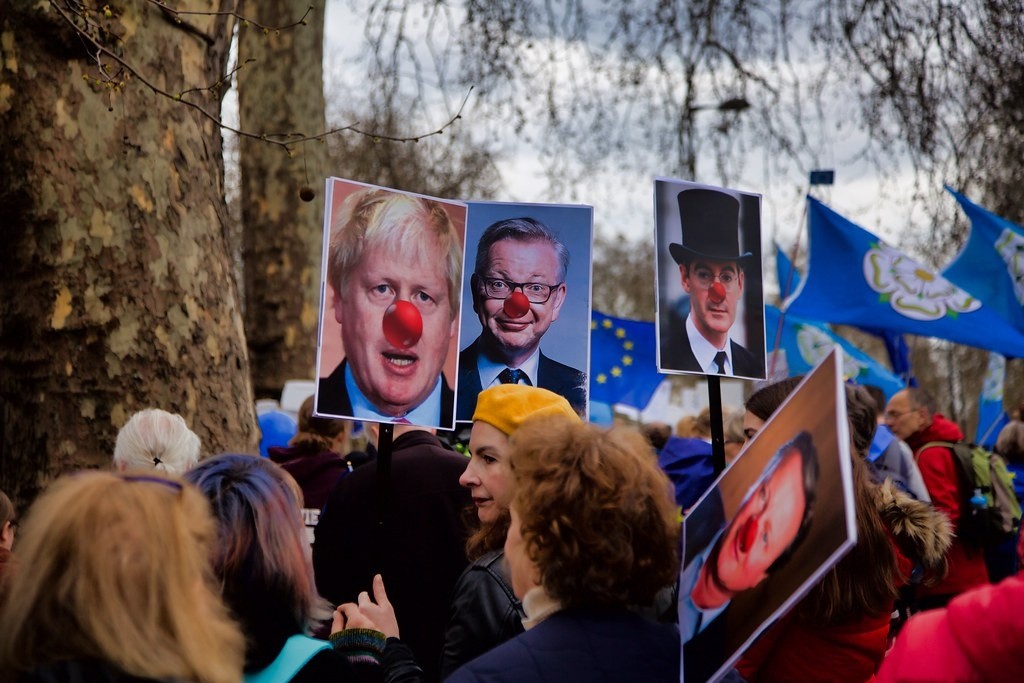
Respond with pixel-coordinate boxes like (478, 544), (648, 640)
(914, 439), (1023, 562)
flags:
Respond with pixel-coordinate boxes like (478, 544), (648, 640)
(775, 246), (804, 300)
(589, 311), (666, 411)
(975, 353), (1009, 447)
(753, 299), (905, 411)
(942, 184), (1024, 335)
(779, 196), (1023, 358)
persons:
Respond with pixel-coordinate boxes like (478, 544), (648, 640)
(457, 216), (586, 420)
(0, 383), (1024, 683)
(664, 189), (761, 378)
(679, 431), (820, 683)
(317, 189), (463, 429)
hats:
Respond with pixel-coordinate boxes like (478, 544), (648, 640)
(257, 411), (298, 459)
(669, 189), (753, 265)
(472, 384), (583, 438)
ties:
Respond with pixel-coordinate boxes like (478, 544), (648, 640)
(713, 351), (726, 375)
(497, 367), (524, 384)
(390, 418), (412, 425)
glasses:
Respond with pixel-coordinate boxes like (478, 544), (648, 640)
(689, 270), (737, 282)
(476, 273), (563, 304)
